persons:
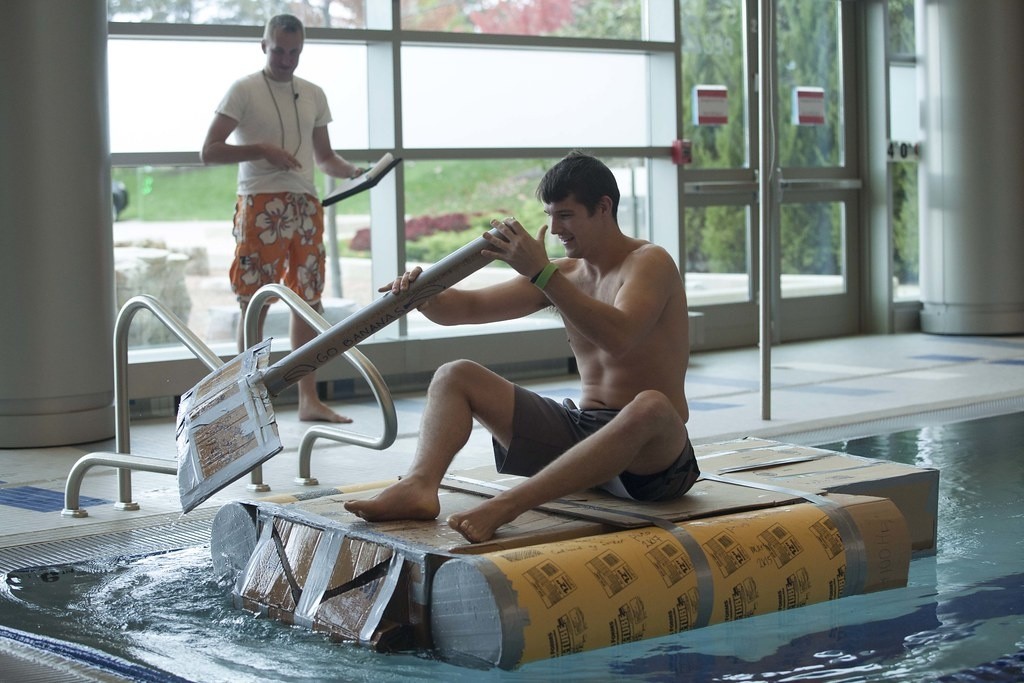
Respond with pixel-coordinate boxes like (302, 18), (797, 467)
(202, 14), (367, 424)
(343, 150), (701, 544)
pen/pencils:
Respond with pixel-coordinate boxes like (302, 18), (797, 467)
(351, 167), (373, 180)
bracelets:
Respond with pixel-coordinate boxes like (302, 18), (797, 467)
(529, 261), (557, 290)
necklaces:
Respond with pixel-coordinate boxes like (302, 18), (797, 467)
(262, 70), (302, 160)
